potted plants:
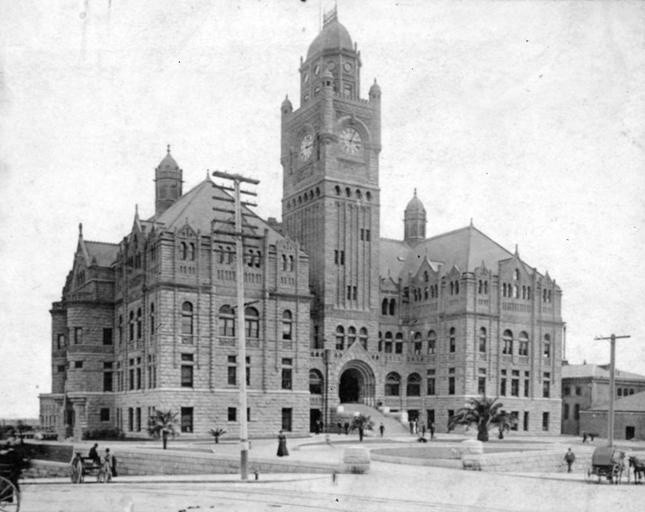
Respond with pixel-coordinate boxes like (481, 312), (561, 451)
(208, 429), (226, 443)
(148, 410), (181, 450)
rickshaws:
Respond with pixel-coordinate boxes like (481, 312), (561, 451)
(1, 440), (32, 512)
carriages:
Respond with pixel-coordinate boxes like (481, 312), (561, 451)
(586, 445), (631, 484)
(70, 453), (111, 485)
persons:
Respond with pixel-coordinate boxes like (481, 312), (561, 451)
(582, 431), (587, 444)
(275, 429), (289, 456)
(314, 413), (438, 443)
(588, 432), (594, 443)
(88, 442), (102, 467)
(103, 447), (114, 483)
(72, 451), (87, 475)
(90, 457), (104, 483)
(563, 446), (576, 473)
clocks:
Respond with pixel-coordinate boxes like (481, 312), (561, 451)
(338, 126), (363, 155)
(298, 133), (314, 161)
